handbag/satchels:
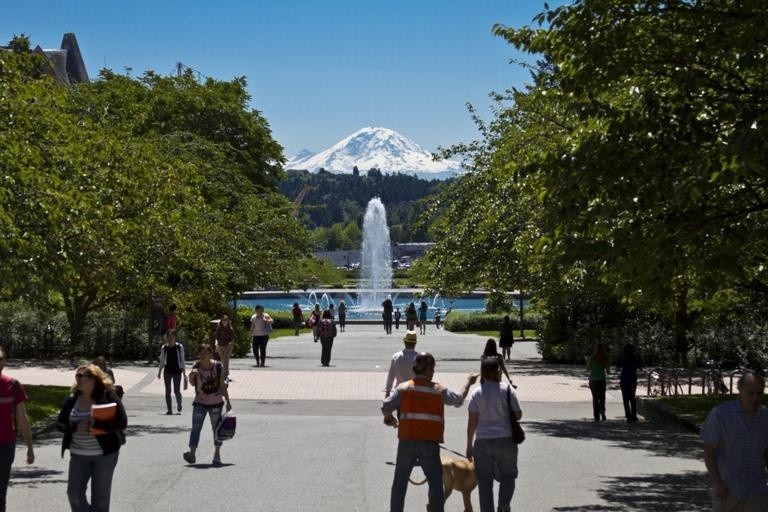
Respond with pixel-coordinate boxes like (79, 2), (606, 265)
(506, 387), (526, 444)
(213, 409), (238, 444)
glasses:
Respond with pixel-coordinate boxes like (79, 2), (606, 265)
(74, 373), (95, 379)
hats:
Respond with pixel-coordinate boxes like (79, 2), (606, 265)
(401, 331), (422, 346)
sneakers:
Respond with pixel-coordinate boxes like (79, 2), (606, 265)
(183, 452), (222, 468)
(593, 416), (639, 422)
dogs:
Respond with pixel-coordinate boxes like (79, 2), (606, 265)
(427, 457), (479, 512)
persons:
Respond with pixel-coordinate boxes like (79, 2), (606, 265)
(381, 299), (428, 335)
(701, 371), (768, 512)
(144, 304), (188, 414)
(338, 301), (347, 332)
(445, 307), (451, 316)
(216, 314), (234, 381)
(381, 353), (479, 512)
(498, 316), (514, 360)
(467, 356), (521, 512)
(309, 302), (337, 366)
(481, 339), (517, 390)
(616, 344), (643, 422)
(182, 344), (231, 467)
(584, 343), (609, 421)
(293, 303), (304, 335)
(385, 330), (419, 398)
(434, 308), (440, 329)
(249, 304), (274, 367)
(56, 365), (128, 512)
(0, 346), (35, 512)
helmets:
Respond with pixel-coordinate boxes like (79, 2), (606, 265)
(412, 353), (435, 372)
